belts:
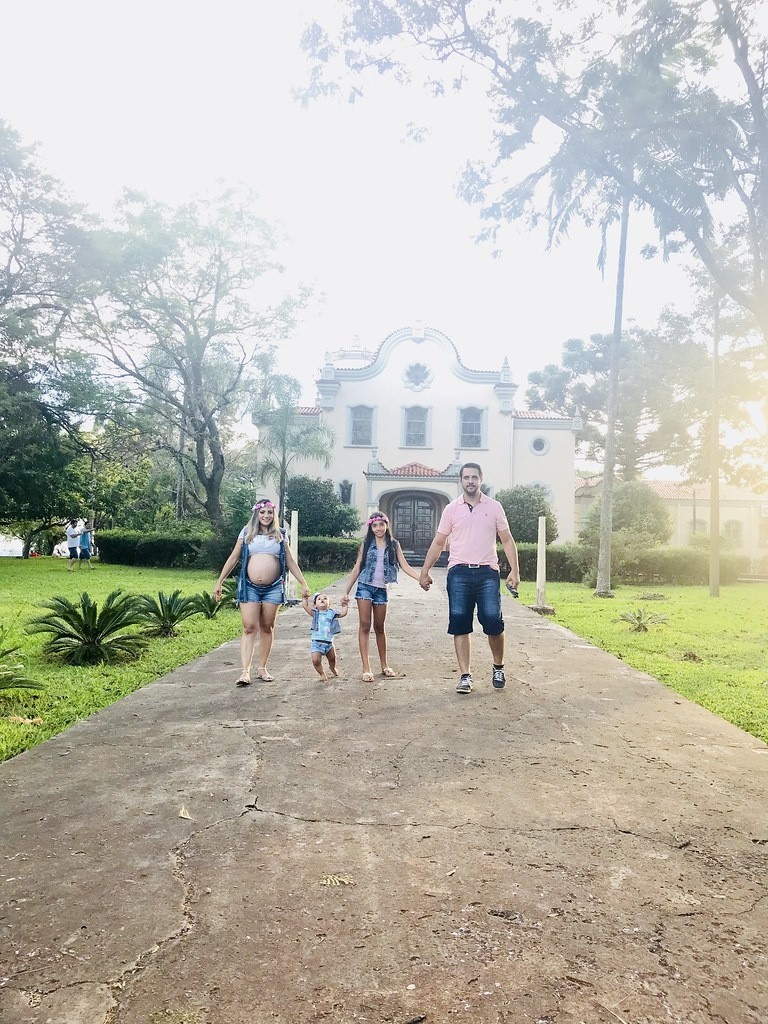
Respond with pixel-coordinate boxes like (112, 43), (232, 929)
(458, 563), (490, 568)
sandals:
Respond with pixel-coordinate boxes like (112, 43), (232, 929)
(235, 670), (251, 684)
(256, 668), (274, 681)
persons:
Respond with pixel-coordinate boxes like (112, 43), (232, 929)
(302, 593), (348, 682)
(341, 511), (430, 681)
(214, 498), (311, 686)
(419, 462), (521, 693)
(67, 520), (95, 572)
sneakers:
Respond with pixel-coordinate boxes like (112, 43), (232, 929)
(492, 663), (506, 689)
(457, 674), (473, 692)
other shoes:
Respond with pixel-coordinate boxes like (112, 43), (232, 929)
(506, 584), (519, 598)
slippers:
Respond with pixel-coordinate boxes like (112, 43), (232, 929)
(362, 673), (374, 682)
(382, 668), (396, 677)
